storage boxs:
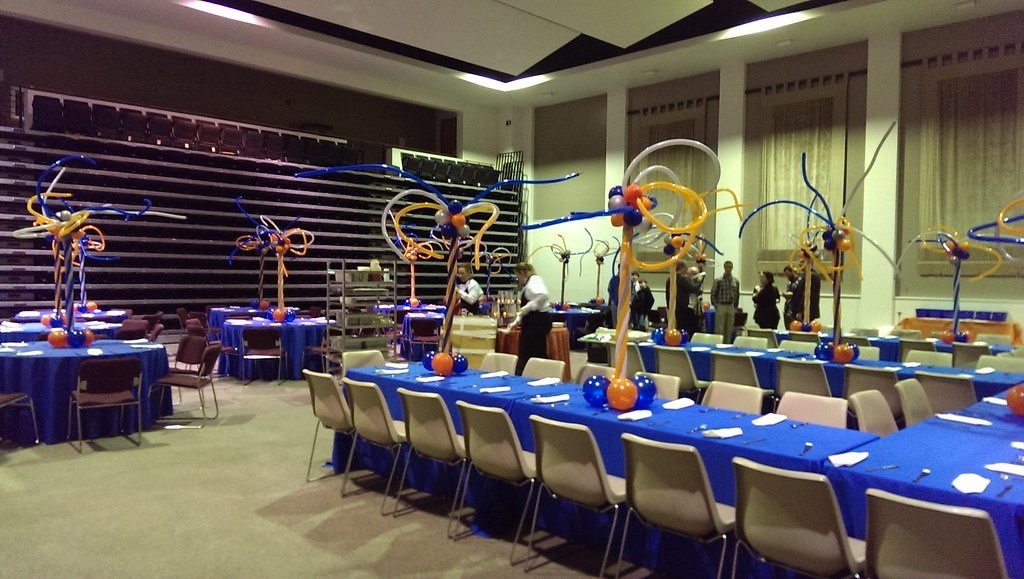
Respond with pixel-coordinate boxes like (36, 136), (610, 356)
(329, 335), (388, 351)
(336, 311), (380, 328)
(334, 270), (381, 283)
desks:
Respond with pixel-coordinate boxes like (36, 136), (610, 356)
(636, 341), (1024, 402)
(335, 359), (880, 579)
(663, 309), (737, 345)
(823, 384), (1024, 579)
(904, 318), (1020, 345)
(775, 330), (1012, 363)
(15, 310), (129, 323)
(0, 340), (168, 445)
(0, 322), (122, 343)
(402, 313), (445, 361)
(224, 318), (337, 380)
(550, 308), (601, 350)
(374, 305), (446, 345)
(210, 307), (301, 342)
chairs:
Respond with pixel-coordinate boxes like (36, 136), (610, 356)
(0, 303), (289, 463)
(297, 299), (1024, 579)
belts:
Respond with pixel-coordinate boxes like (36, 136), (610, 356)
(718, 301), (734, 305)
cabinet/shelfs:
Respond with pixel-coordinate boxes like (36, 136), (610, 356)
(327, 257), (398, 377)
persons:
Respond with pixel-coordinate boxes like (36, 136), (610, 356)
(665, 260), (701, 331)
(608, 264), (636, 330)
(793, 257), (821, 324)
(454, 264), (484, 315)
(686, 266), (704, 331)
(779, 266), (801, 330)
(631, 280), (655, 330)
(629, 270), (641, 295)
(710, 260), (740, 345)
(503, 263), (551, 376)
(752, 271), (782, 330)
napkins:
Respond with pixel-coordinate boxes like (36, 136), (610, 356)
(480, 371), (510, 379)
(982, 397), (1008, 406)
(530, 393), (570, 404)
(745, 351), (765, 357)
(662, 397), (695, 410)
(385, 361), (409, 369)
(951, 473), (992, 495)
(827, 451), (869, 468)
(902, 362), (922, 368)
(752, 412), (788, 427)
(617, 409), (653, 422)
(479, 386), (511, 394)
(974, 367), (997, 374)
(985, 462), (1024, 477)
(415, 376), (446, 384)
(375, 368), (410, 375)
(527, 377), (561, 387)
(935, 412), (993, 426)
(702, 427), (743, 439)
(1012, 442), (1024, 449)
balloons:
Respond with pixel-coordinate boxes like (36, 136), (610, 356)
(293, 122), (1024, 417)
(227, 196), (315, 323)
(12, 155), (187, 348)
